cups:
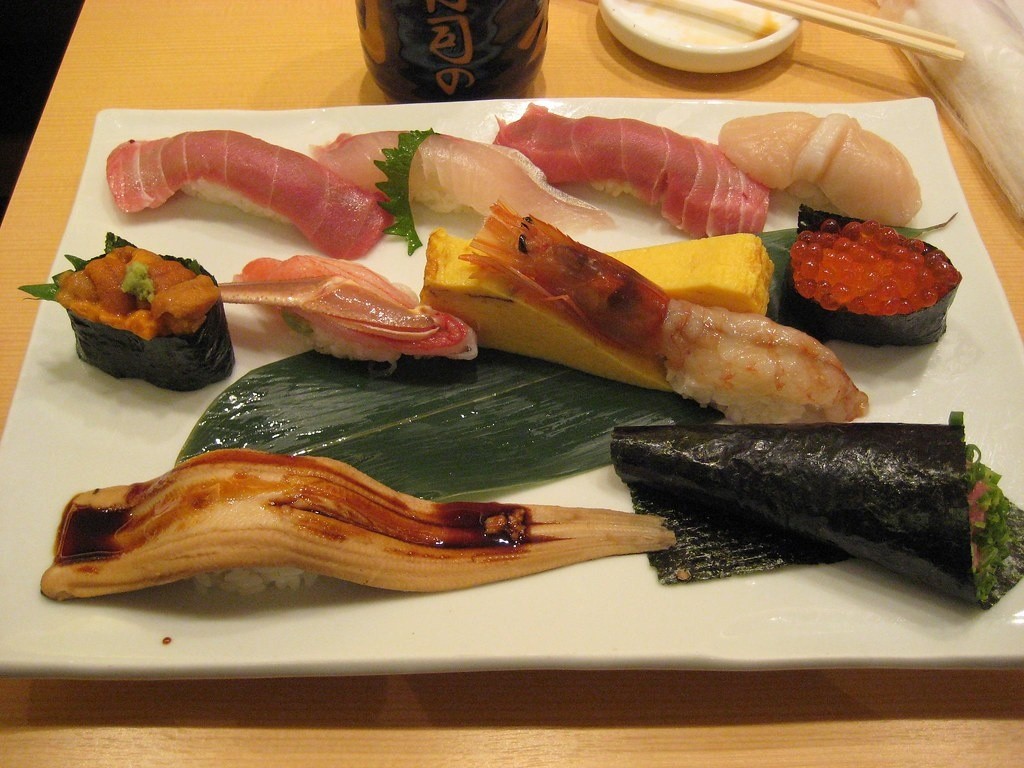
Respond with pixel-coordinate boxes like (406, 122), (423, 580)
(355, 0), (549, 103)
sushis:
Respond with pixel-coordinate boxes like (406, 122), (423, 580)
(10, 106), (1024, 607)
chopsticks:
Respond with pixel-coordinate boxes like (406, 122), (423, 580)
(751, 0), (965, 63)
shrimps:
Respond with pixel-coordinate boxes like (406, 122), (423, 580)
(456, 197), (867, 421)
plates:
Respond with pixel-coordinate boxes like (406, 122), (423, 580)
(598, 0), (801, 72)
(0, 98), (1024, 673)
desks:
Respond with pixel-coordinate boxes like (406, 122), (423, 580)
(0, 1), (1024, 768)
